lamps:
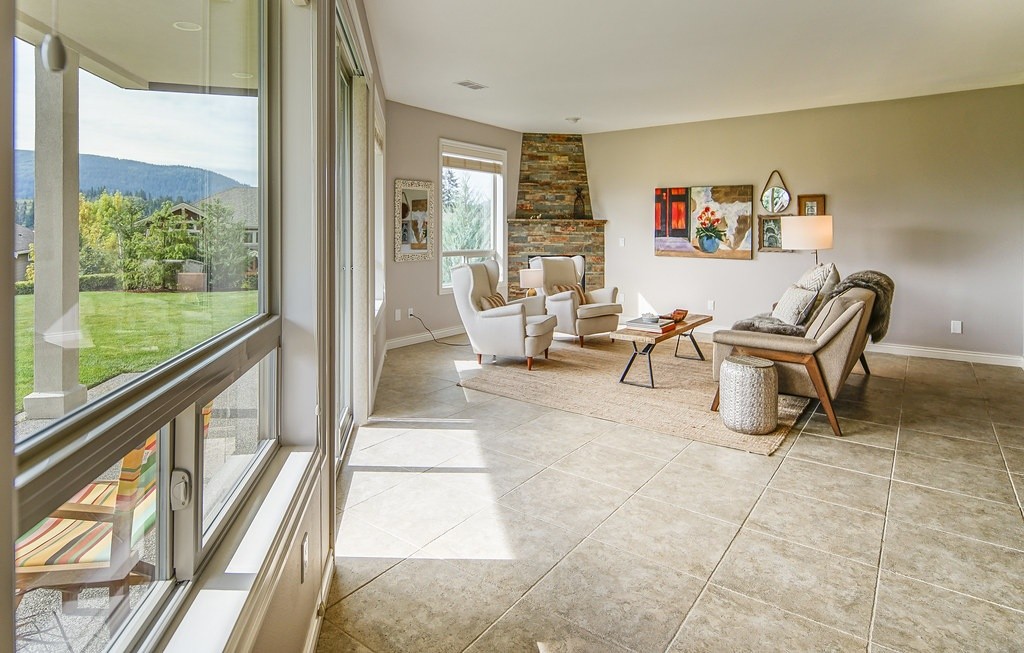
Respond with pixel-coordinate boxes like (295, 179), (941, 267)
(781, 215), (833, 264)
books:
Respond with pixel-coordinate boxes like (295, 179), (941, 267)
(626, 316), (675, 333)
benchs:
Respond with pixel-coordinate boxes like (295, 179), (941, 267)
(14, 399), (213, 641)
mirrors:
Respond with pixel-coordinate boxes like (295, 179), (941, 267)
(393, 178), (435, 262)
(760, 185), (791, 213)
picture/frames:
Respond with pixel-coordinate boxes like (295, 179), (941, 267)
(757, 214), (800, 254)
(796, 193), (825, 215)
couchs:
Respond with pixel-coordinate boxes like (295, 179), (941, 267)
(449, 260), (557, 370)
(527, 253), (624, 347)
(708, 270), (895, 437)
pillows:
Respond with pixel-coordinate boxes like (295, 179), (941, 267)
(479, 291), (507, 310)
(769, 282), (819, 326)
(553, 282), (586, 305)
(795, 260), (842, 327)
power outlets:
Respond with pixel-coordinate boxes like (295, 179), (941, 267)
(408, 307), (414, 318)
(707, 299), (715, 311)
(951, 320), (961, 333)
(394, 309), (401, 321)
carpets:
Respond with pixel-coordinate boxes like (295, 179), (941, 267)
(455, 330), (812, 456)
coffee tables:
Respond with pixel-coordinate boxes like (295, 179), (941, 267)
(609, 313), (713, 387)
